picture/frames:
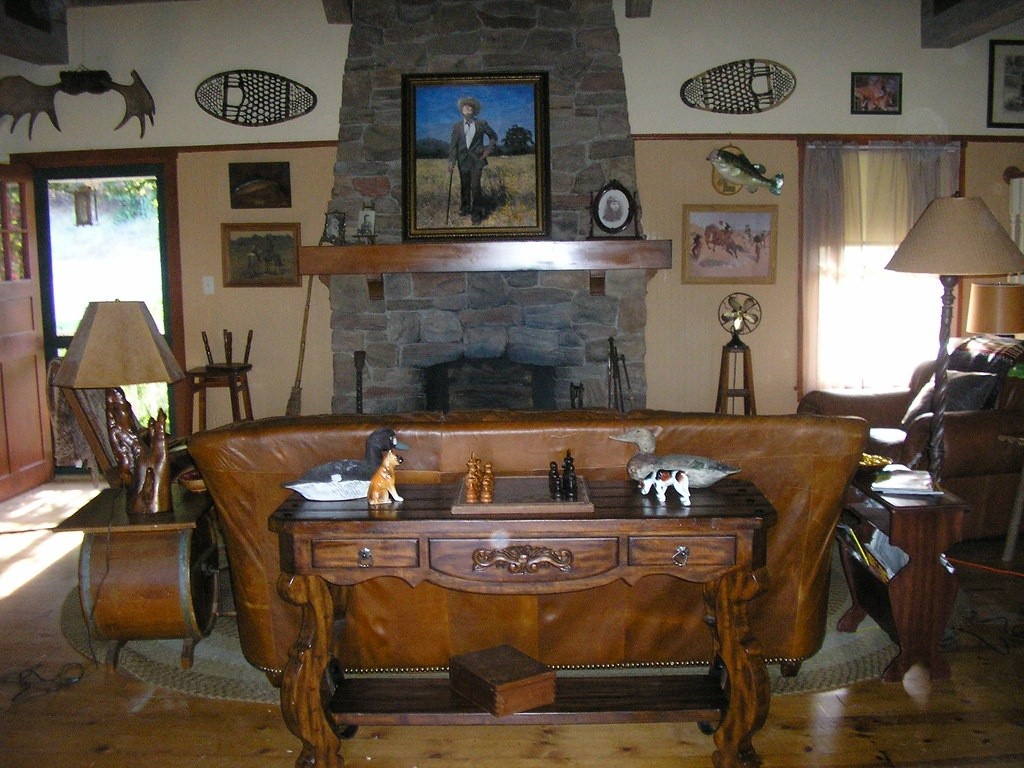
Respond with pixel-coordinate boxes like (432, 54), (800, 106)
(681, 204), (779, 285)
(987, 39), (1024, 128)
(593, 182), (633, 234)
(222, 223), (303, 288)
(325, 212), (347, 241)
(851, 71), (903, 115)
(398, 72), (552, 240)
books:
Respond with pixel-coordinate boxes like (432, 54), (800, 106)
(834, 520), (907, 584)
(870, 464), (943, 496)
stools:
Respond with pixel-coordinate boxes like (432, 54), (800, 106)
(200, 328), (255, 371)
(185, 369), (254, 438)
(716, 349), (758, 415)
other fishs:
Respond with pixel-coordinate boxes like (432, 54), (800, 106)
(707, 147), (784, 196)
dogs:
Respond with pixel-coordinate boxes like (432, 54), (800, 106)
(366, 450), (404, 506)
(639, 469), (692, 508)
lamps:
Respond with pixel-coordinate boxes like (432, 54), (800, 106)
(884, 195), (1024, 489)
(50, 300), (188, 513)
(72, 185), (99, 226)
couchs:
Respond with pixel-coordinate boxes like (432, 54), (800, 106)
(798, 361), (1024, 546)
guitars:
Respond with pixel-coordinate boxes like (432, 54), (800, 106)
(354, 350), (366, 415)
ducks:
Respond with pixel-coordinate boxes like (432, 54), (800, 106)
(610, 429), (742, 489)
(279, 426), (411, 502)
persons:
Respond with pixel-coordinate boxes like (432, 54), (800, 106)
(326, 215), (342, 242)
(602, 196), (622, 222)
(358, 212), (372, 234)
(446, 97), (499, 225)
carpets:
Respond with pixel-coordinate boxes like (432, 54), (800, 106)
(62, 554), (973, 718)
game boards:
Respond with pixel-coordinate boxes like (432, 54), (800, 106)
(450, 473), (594, 514)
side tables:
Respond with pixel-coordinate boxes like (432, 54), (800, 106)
(829, 471), (969, 684)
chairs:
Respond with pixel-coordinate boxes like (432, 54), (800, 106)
(46, 357), (229, 551)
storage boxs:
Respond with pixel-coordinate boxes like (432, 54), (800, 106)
(446, 646), (554, 720)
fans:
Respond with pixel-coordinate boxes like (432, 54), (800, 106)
(716, 292), (762, 351)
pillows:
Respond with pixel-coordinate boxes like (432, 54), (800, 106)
(898, 337), (1013, 426)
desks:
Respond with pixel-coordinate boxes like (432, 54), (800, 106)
(48, 485), (218, 673)
(267, 480), (779, 767)
